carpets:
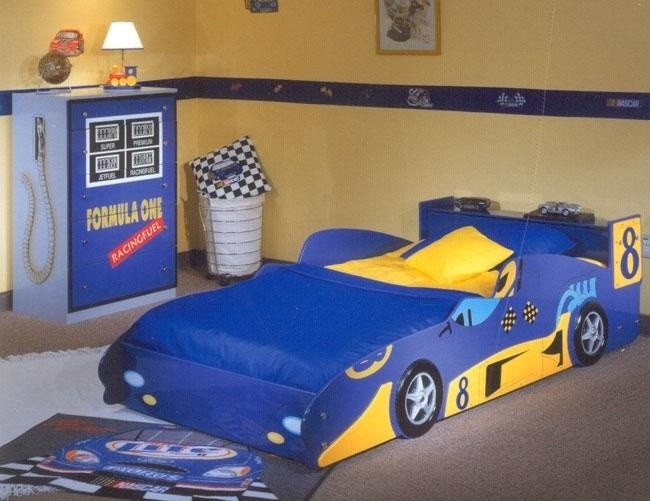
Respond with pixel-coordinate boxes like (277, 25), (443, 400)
(0, 413), (336, 501)
(0, 343), (175, 447)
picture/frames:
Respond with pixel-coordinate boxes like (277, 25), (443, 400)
(374, 0), (441, 56)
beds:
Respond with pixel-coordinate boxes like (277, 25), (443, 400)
(98, 195), (644, 470)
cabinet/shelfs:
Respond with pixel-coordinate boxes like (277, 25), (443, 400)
(12, 87), (178, 325)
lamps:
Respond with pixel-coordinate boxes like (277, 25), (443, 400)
(101, 21), (143, 90)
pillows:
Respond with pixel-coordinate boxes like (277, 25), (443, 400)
(487, 220), (578, 268)
(400, 223), (515, 286)
(188, 137), (272, 198)
